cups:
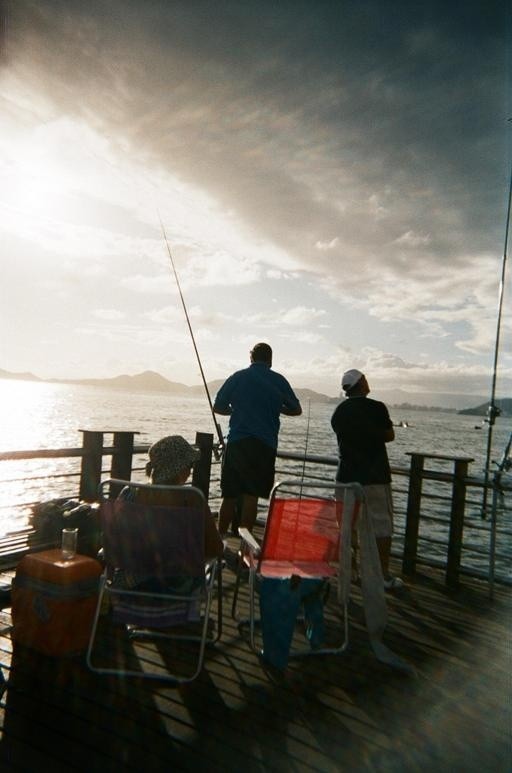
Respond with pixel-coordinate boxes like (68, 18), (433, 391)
(61, 527), (80, 559)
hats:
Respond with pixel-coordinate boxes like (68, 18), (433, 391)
(148, 435), (203, 469)
(341, 369), (364, 391)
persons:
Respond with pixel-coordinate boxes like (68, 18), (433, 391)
(112, 436), (224, 636)
(331, 370), (405, 588)
(212, 343), (302, 545)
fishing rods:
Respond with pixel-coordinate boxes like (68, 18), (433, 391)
(490, 431), (512, 484)
(289, 398), (310, 563)
(475, 176), (512, 519)
(155, 203), (226, 460)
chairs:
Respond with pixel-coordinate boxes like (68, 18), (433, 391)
(86, 479), (228, 683)
(232, 482), (365, 658)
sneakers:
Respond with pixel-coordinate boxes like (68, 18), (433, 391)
(383, 575), (405, 588)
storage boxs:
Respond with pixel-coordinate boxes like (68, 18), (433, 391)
(12, 549), (103, 657)
